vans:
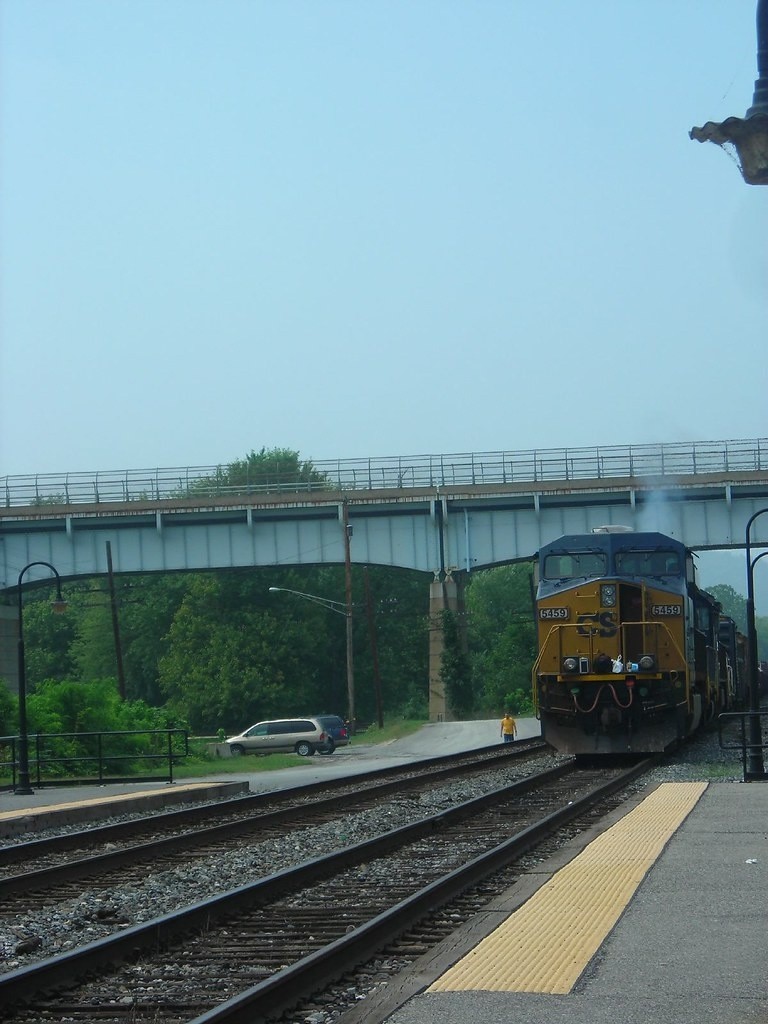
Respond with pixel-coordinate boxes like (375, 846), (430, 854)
(223, 718), (329, 758)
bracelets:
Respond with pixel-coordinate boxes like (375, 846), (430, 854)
(515, 731), (517, 732)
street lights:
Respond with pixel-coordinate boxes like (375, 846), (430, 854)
(16, 562), (67, 795)
(267, 587), (356, 735)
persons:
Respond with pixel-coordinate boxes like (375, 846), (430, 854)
(500, 713), (517, 743)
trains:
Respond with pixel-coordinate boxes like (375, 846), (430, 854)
(530, 523), (768, 759)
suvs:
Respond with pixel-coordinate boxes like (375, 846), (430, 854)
(255, 715), (351, 757)
